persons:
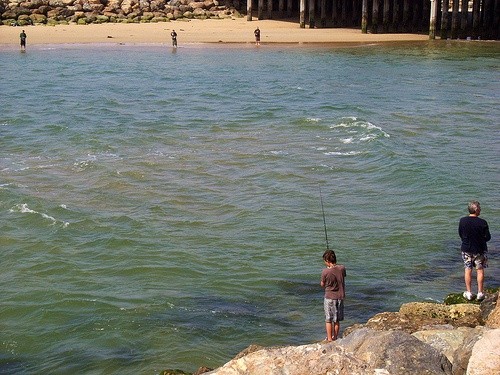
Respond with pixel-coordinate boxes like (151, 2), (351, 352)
(171, 30), (177, 48)
(320, 250), (347, 342)
(20, 30), (27, 51)
(254, 27), (261, 46)
(458, 201), (491, 300)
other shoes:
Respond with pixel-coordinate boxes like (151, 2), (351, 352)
(463, 292), (472, 300)
(321, 338), (331, 344)
(477, 294), (484, 300)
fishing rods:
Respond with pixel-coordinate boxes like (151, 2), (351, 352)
(318, 184), (328, 250)
